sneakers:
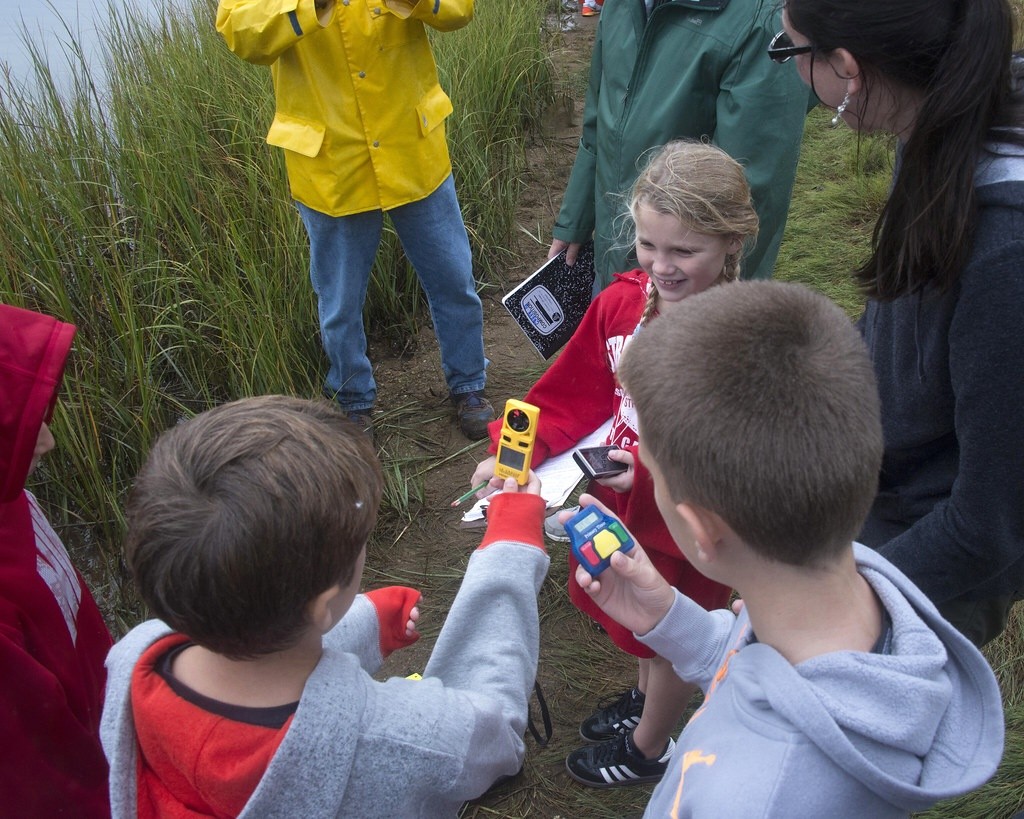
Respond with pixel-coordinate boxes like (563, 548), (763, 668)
(448, 390), (496, 441)
(579, 685), (645, 743)
(581, 2), (602, 17)
(565, 732), (675, 788)
(347, 408), (374, 441)
(544, 505), (580, 543)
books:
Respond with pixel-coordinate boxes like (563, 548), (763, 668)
(502, 237), (594, 361)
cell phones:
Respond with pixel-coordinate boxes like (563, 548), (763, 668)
(572, 444), (628, 480)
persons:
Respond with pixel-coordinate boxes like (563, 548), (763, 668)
(578, 0), (604, 16)
(99, 393), (550, 819)
(560, 281), (1004, 819)
(0, 301), (114, 819)
(548, 0), (819, 301)
(472, 142), (759, 788)
(732, 0), (1024, 651)
(215, 0), (496, 440)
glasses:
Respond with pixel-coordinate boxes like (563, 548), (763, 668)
(767, 28), (835, 64)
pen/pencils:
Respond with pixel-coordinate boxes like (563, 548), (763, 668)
(450, 480), (489, 507)
